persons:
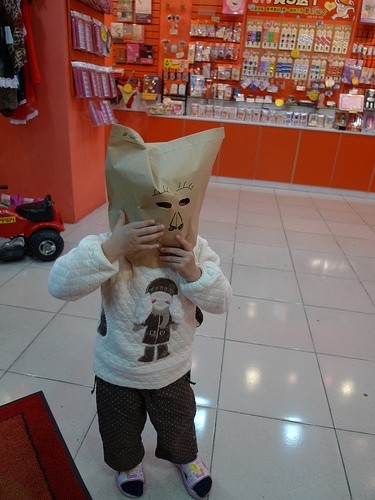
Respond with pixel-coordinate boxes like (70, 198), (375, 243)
(47, 122), (233, 500)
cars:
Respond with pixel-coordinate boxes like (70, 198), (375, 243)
(0, 184), (64, 264)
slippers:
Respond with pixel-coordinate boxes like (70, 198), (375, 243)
(177, 455), (212, 499)
(116, 461), (145, 498)
